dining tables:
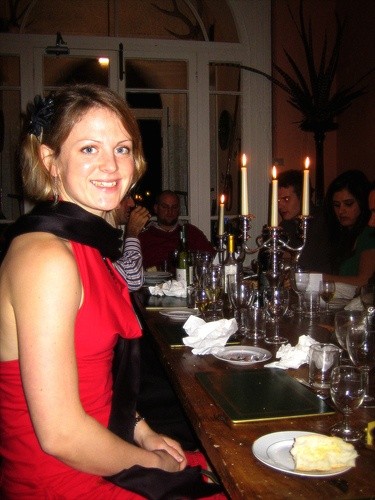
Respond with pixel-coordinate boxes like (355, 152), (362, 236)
(131, 278), (375, 500)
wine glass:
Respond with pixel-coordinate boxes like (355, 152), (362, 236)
(192, 256), (224, 323)
(229, 281), (254, 335)
(262, 288), (289, 344)
(318, 280), (335, 314)
(291, 271), (309, 314)
(328, 365), (368, 442)
(334, 310), (375, 408)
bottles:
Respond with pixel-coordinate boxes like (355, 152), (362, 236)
(170, 216), (194, 286)
(221, 235), (239, 309)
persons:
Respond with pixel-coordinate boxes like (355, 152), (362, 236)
(0, 83), (226, 500)
(257, 169), (375, 312)
(136, 190), (214, 271)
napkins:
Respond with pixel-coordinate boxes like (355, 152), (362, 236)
(148, 279), (192, 299)
(263, 335), (321, 369)
(344, 296), (365, 311)
(183, 315), (238, 356)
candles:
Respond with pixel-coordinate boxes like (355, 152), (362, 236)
(219, 194), (224, 235)
(302, 156), (311, 216)
(241, 154), (250, 215)
(270, 166), (279, 227)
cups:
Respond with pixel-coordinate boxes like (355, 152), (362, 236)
(244, 307), (267, 340)
(303, 291), (320, 318)
(307, 343), (342, 400)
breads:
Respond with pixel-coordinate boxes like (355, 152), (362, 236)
(291, 434), (359, 472)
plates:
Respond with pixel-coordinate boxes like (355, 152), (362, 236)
(251, 430), (355, 477)
(159, 308), (201, 320)
(213, 345), (272, 366)
(144, 271), (173, 279)
